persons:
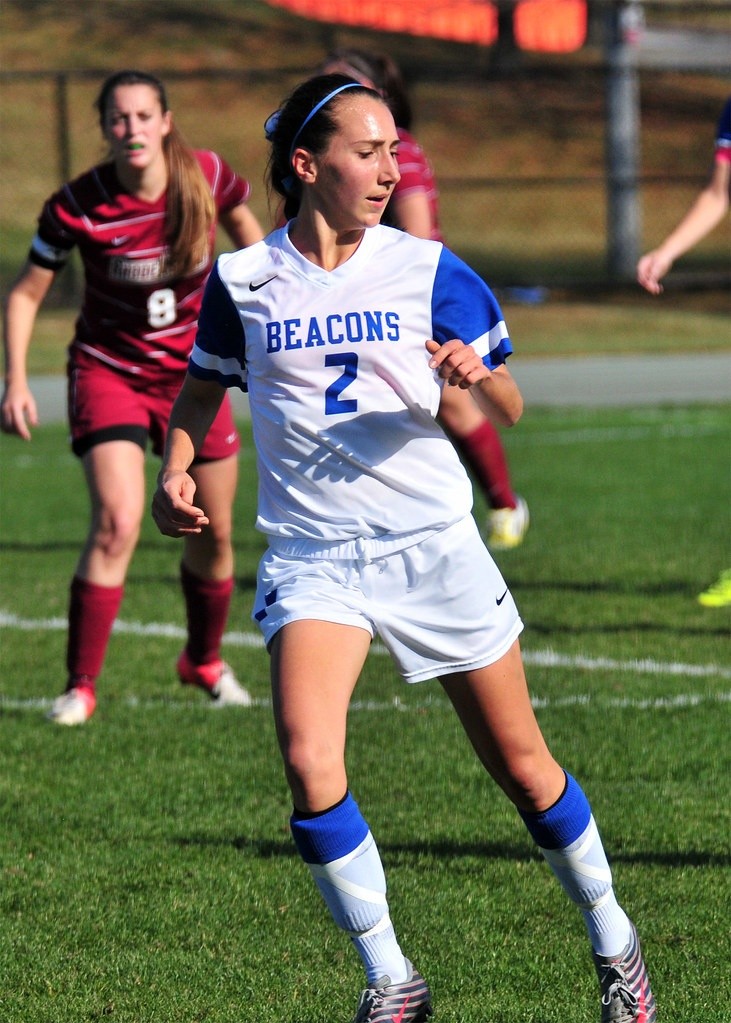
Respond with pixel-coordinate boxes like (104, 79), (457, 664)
(148, 65), (659, 1023)
(634, 83), (731, 609)
(0, 68), (272, 727)
(264, 48), (531, 553)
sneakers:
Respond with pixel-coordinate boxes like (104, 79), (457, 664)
(696, 566), (731, 610)
(590, 918), (658, 1023)
(46, 684), (98, 728)
(487, 492), (531, 553)
(357, 956), (434, 1022)
(176, 648), (253, 705)
